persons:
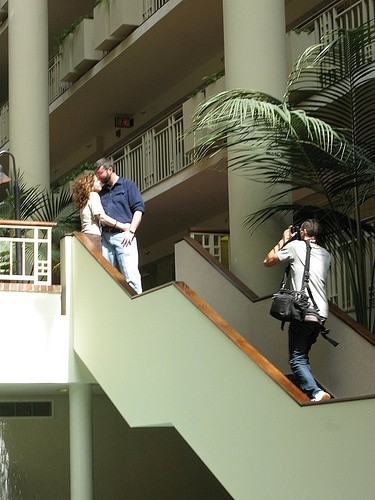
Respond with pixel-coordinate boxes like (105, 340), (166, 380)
(72, 170), (131, 256)
(263, 219), (331, 402)
(95, 158), (145, 295)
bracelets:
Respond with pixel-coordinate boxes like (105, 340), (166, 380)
(114, 221), (117, 228)
(129, 230), (135, 234)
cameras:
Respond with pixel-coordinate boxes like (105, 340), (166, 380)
(291, 225), (300, 240)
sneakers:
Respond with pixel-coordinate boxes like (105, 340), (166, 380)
(311, 390), (331, 401)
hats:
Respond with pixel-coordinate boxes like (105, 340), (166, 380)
(0, 164), (11, 184)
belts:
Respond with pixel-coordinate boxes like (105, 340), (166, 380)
(103, 227), (124, 234)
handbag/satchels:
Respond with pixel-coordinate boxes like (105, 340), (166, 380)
(270, 289), (311, 330)
